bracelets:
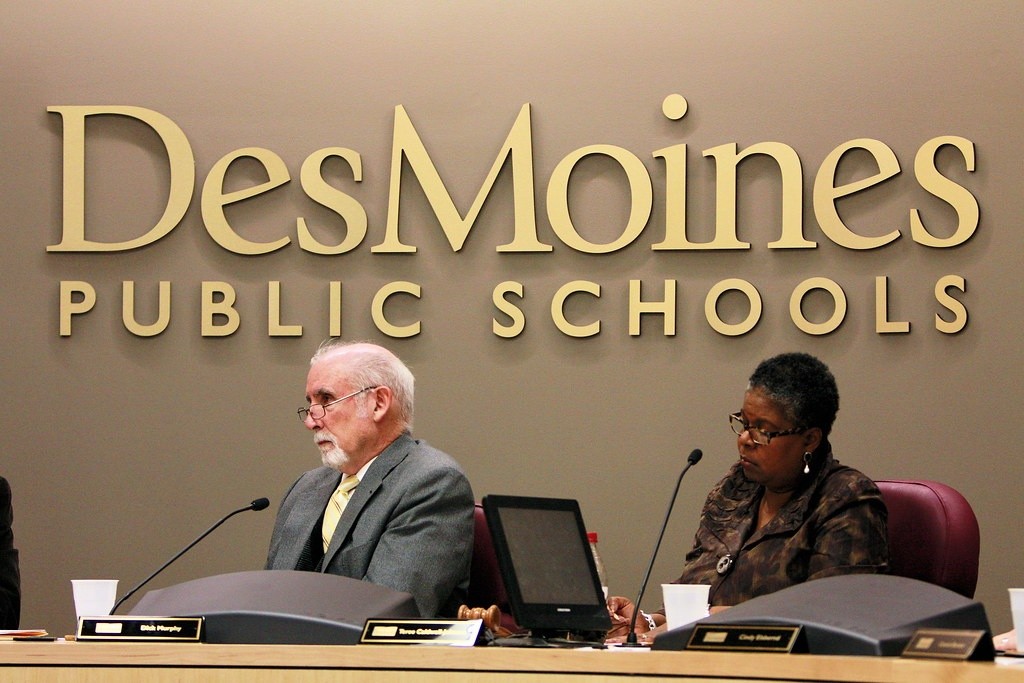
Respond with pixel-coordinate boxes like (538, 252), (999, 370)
(639, 608), (656, 631)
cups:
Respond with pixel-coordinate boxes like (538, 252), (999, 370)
(1007, 588), (1023, 651)
(660, 583), (712, 632)
(71, 579), (120, 623)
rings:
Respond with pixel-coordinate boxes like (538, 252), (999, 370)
(642, 631), (648, 639)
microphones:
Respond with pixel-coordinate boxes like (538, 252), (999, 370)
(618, 448), (704, 648)
(109, 498), (271, 616)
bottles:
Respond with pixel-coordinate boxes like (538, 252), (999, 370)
(587, 531), (609, 607)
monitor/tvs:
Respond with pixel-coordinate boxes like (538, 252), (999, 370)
(482, 494), (614, 650)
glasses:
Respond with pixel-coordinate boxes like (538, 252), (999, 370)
(728, 411), (810, 445)
(296, 386), (377, 423)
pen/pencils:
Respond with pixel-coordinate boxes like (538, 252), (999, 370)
(13, 638), (54, 641)
(607, 605), (621, 623)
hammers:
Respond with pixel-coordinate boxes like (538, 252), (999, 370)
(457, 604), (512, 636)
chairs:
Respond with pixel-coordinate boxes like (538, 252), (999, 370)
(469, 503), (514, 613)
(868, 477), (981, 601)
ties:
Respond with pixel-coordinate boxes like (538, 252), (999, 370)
(322, 475), (360, 555)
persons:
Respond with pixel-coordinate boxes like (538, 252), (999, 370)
(606, 351), (888, 645)
(264, 339), (475, 620)
(0, 477), (21, 630)
